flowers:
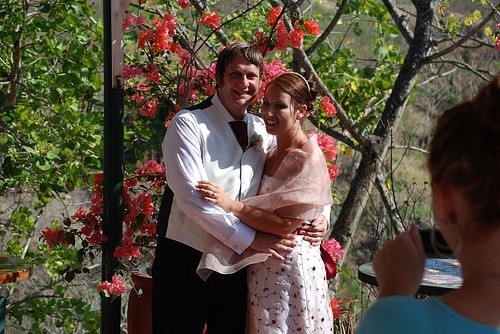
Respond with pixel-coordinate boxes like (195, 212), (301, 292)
(45, 0), (347, 298)
(243, 132), (264, 154)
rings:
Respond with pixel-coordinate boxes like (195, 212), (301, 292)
(211, 192), (215, 198)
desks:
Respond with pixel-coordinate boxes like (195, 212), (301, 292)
(358, 257), (464, 297)
(0, 254), (34, 334)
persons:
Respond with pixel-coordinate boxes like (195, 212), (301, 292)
(353, 78), (500, 334)
(151, 43), (333, 334)
(197, 72), (334, 334)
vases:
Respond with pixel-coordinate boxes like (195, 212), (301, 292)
(127, 266), (208, 334)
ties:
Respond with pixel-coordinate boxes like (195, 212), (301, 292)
(228, 120), (248, 150)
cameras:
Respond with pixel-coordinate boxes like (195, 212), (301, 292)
(420, 229), (454, 259)
